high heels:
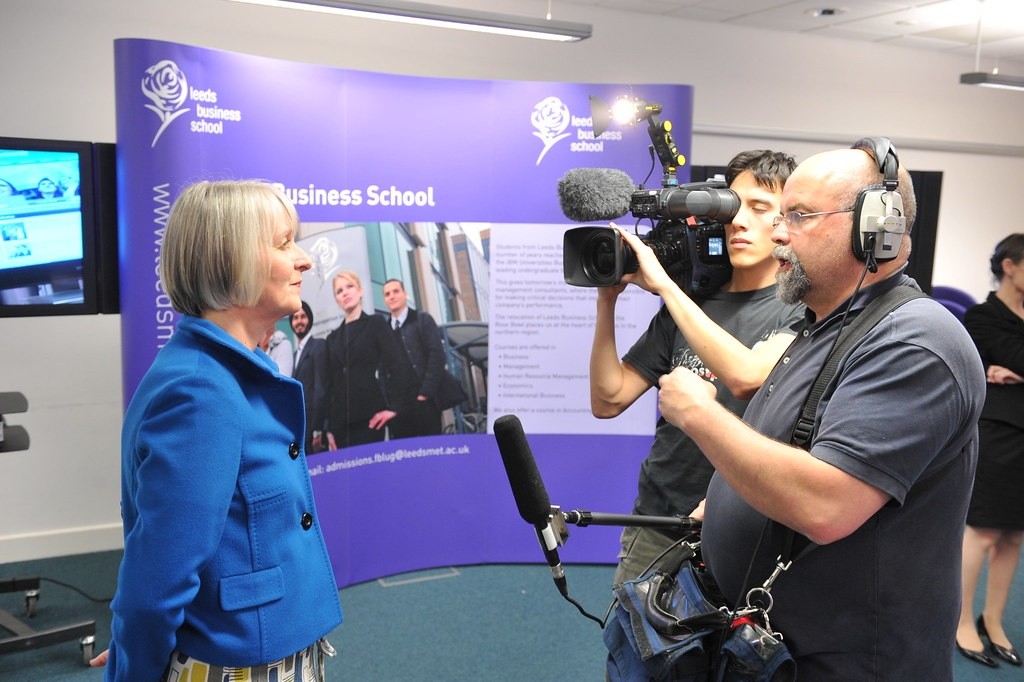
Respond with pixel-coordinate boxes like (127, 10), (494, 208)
(976, 613), (1021, 665)
(956, 640), (999, 668)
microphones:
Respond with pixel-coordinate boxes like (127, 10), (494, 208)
(493, 415), (569, 600)
(559, 166), (639, 222)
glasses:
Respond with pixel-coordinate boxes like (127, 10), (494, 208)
(772, 209), (856, 233)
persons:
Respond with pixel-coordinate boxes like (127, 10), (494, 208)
(0, 180), (15, 196)
(259, 324), (293, 378)
(589, 151), (810, 610)
(90, 179), (344, 682)
(289, 300), (327, 456)
(658, 136), (987, 682)
(323, 271), (413, 451)
(381, 278), (448, 440)
(36, 179), (62, 199)
(955, 234), (1024, 668)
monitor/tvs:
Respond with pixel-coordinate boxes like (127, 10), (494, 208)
(0, 136), (121, 318)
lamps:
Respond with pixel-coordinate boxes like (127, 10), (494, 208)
(227, 0), (593, 44)
(958, 0), (1024, 92)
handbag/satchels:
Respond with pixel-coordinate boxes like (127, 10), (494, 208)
(603, 543), (798, 682)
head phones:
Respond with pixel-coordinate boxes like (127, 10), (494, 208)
(851, 136), (907, 266)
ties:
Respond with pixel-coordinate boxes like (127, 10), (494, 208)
(395, 319), (400, 329)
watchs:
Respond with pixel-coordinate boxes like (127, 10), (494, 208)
(312, 430), (321, 438)
